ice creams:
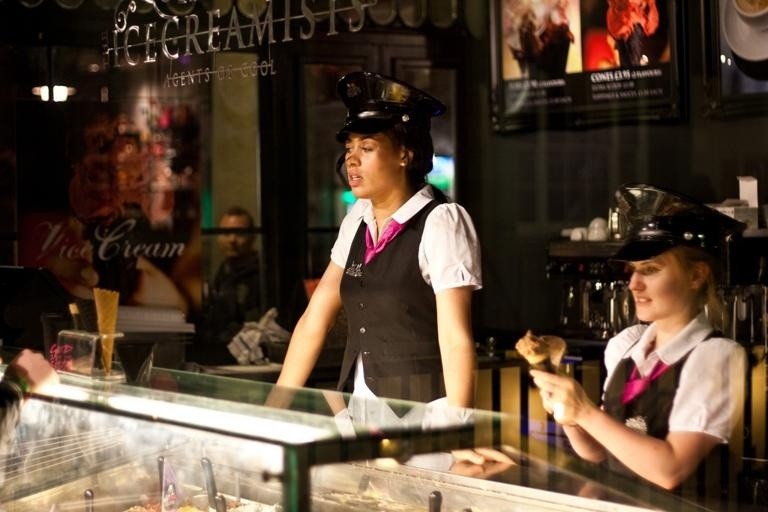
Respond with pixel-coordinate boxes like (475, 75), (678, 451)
(515, 329), (566, 371)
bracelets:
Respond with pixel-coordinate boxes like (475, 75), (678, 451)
(3, 373), (30, 408)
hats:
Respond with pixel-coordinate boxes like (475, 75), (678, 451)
(334, 72), (448, 144)
(608, 183), (747, 261)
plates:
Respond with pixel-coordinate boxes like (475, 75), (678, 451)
(718, 0), (768, 65)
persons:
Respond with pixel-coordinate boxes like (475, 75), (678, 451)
(529, 180), (750, 494)
(1, 342), (61, 474)
(260, 69), (520, 469)
(181, 205), (261, 364)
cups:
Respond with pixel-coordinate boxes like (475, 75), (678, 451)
(731, 0), (768, 19)
(560, 207), (623, 244)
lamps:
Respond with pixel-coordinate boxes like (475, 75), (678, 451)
(30, 46), (79, 105)
